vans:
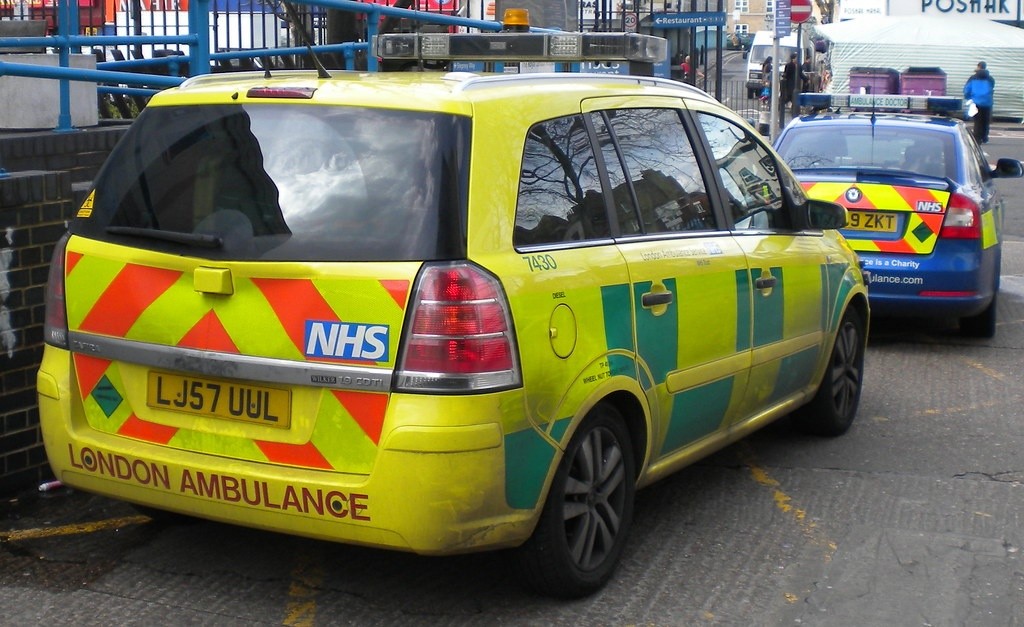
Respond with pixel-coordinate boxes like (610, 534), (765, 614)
(742, 30), (804, 99)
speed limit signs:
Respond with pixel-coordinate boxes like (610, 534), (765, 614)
(625, 13), (637, 28)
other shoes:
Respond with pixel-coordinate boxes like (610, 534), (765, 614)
(761, 105), (770, 109)
(978, 138), (990, 145)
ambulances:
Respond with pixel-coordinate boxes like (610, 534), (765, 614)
(37, 32), (871, 603)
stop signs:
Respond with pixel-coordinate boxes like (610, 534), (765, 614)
(788, 0), (814, 24)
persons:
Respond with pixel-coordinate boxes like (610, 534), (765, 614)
(962, 61), (995, 144)
(758, 55), (812, 110)
(680, 55), (705, 80)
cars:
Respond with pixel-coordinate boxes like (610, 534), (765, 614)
(776, 91), (1024, 335)
